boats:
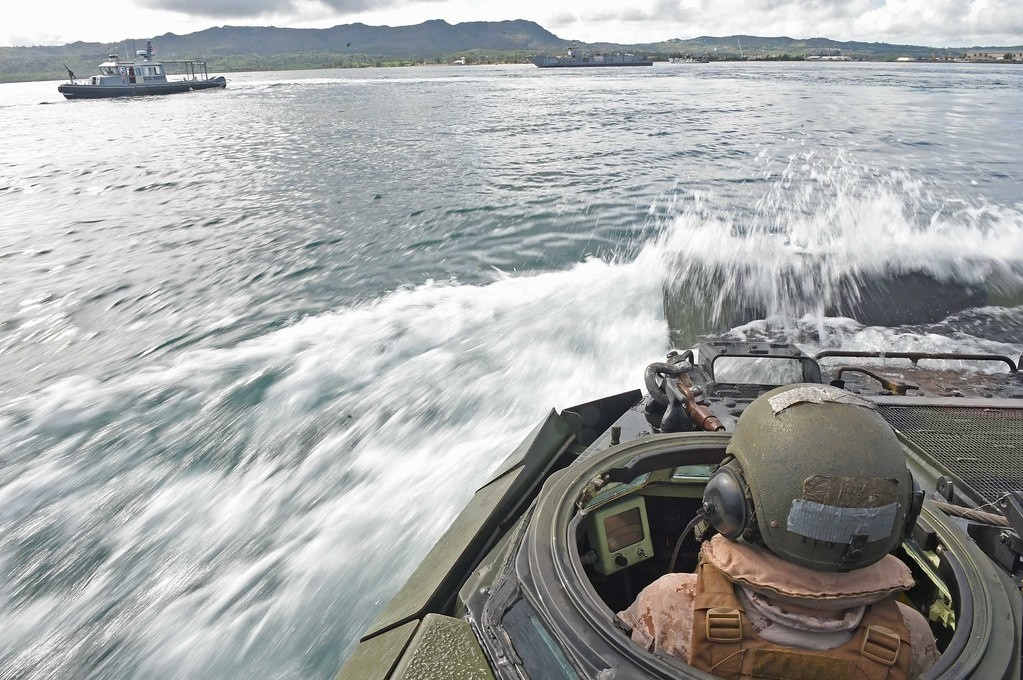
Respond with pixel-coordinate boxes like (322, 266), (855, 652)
(57, 38), (226, 99)
(524, 47), (653, 68)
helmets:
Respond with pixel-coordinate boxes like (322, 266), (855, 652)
(726, 380), (913, 574)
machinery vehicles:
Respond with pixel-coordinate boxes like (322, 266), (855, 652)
(329, 339), (1023, 680)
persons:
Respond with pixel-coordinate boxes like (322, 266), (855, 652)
(118, 68), (125, 75)
(129, 67), (134, 79)
(612, 381), (944, 680)
(107, 67), (111, 74)
